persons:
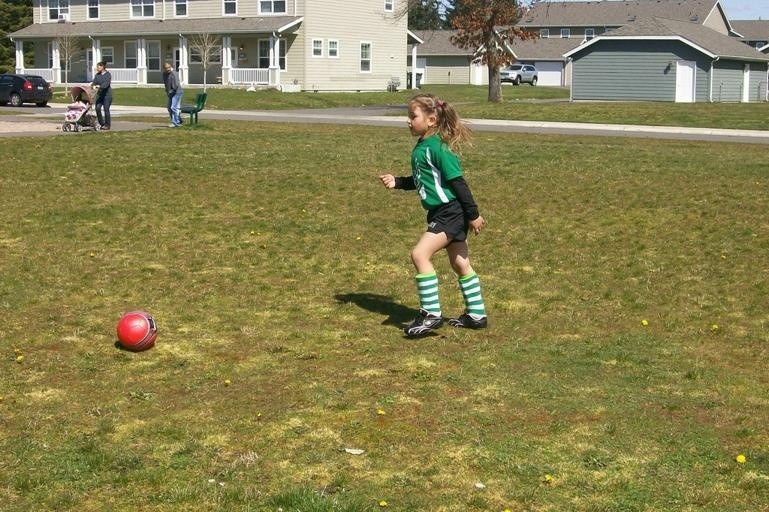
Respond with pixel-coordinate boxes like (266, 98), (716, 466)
(379, 93), (487, 336)
(66, 101), (84, 120)
(163, 62), (183, 127)
(90, 61), (112, 130)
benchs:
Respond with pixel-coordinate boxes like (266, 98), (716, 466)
(180, 94), (208, 125)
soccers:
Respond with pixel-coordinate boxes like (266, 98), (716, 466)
(117, 311), (157, 351)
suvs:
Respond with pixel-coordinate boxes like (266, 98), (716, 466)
(498, 64), (541, 87)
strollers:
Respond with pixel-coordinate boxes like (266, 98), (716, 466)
(59, 84), (101, 133)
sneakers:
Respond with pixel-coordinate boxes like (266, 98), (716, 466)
(405, 308), (442, 335)
(448, 314), (487, 329)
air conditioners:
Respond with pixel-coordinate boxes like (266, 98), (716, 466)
(58, 14), (66, 20)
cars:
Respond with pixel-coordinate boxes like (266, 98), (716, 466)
(0, 73), (53, 106)
